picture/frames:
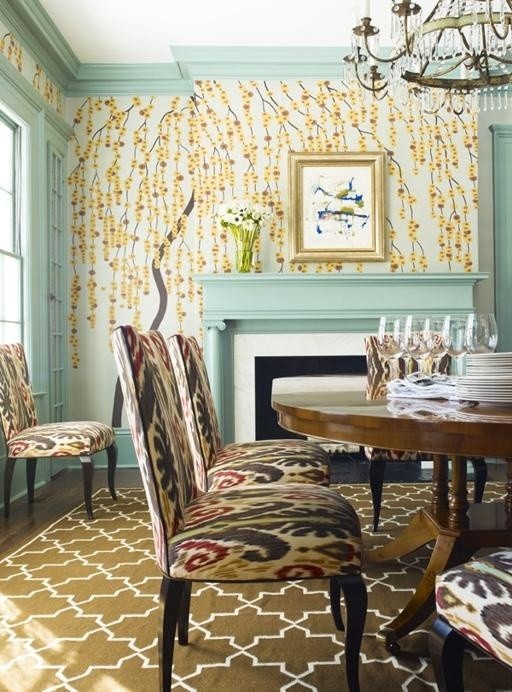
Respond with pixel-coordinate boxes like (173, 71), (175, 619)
(287, 151), (386, 263)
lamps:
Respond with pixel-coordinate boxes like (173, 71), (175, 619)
(343, 0), (512, 103)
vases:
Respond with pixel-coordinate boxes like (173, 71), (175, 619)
(233, 240), (255, 275)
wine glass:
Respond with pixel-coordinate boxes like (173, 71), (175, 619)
(375, 310), (500, 382)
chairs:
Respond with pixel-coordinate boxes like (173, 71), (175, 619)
(0, 342), (119, 520)
(427, 546), (512, 692)
(108, 326), (368, 691)
(163, 333), (331, 492)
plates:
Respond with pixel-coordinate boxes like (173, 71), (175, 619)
(453, 350), (512, 406)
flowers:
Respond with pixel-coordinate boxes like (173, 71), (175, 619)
(216, 202), (275, 271)
